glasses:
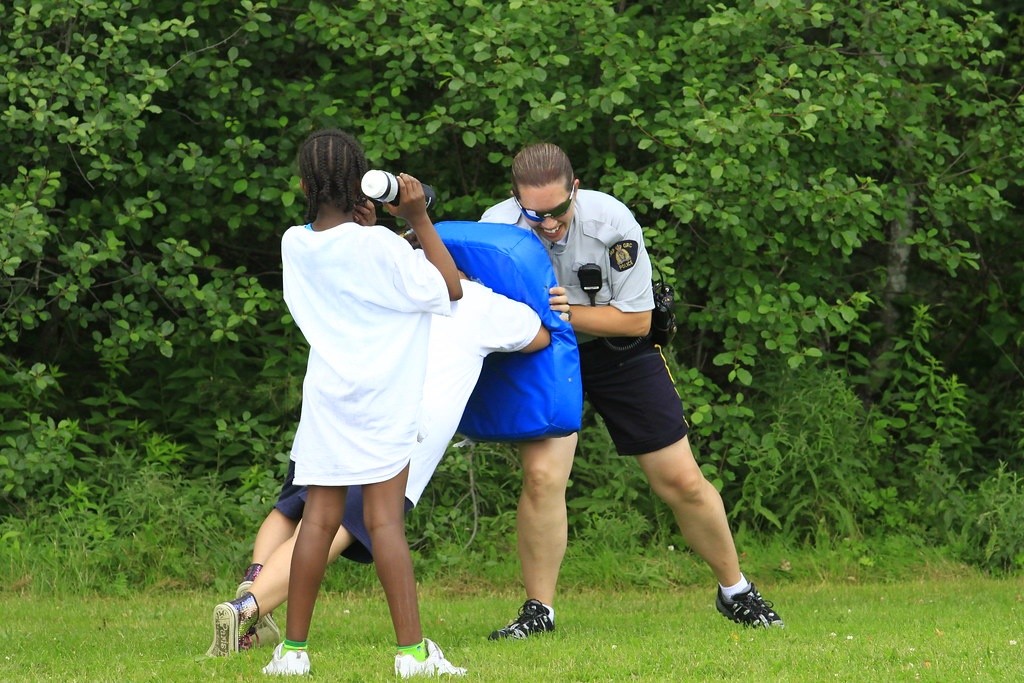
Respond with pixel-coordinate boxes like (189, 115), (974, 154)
(514, 184), (574, 222)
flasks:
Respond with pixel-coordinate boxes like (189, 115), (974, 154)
(361, 169), (436, 213)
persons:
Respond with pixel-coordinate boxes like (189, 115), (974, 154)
(476, 142), (783, 641)
(258, 129), (466, 677)
(199, 230), (547, 661)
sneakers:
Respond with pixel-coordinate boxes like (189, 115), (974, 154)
(395, 638), (468, 678)
(261, 642), (310, 675)
(206, 592), (259, 658)
(488, 601), (555, 640)
(716, 584), (786, 630)
(236, 564), (273, 629)
